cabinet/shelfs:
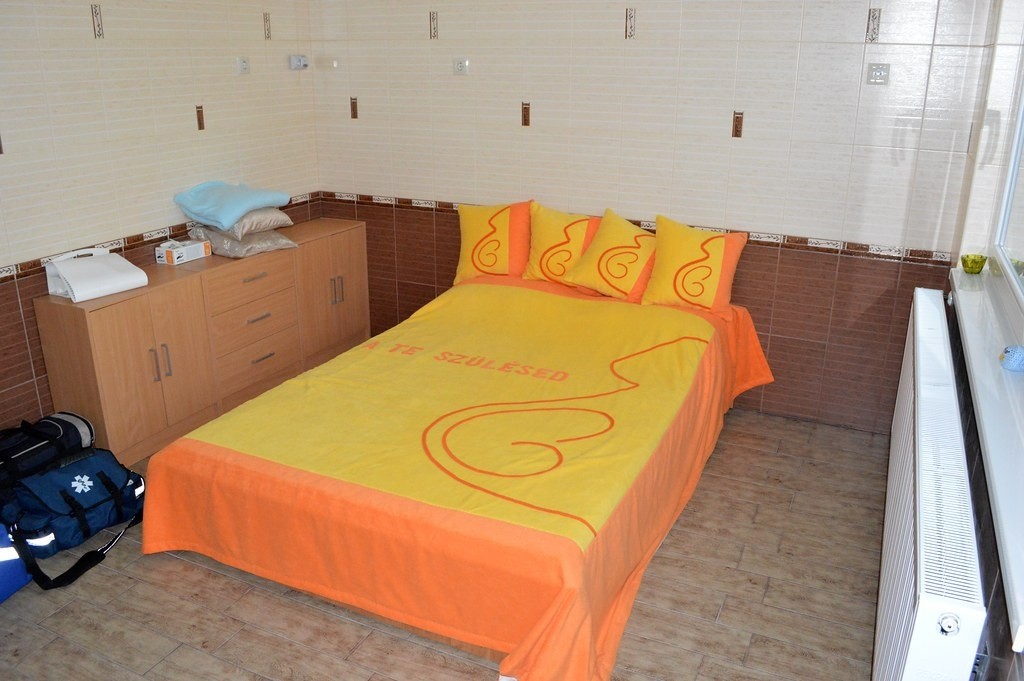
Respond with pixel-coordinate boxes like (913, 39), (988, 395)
(32, 216), (371, 467)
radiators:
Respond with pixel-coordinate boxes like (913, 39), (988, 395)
(873, 288), (987, 681)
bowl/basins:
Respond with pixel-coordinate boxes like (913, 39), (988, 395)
(961, 254), (988, 274)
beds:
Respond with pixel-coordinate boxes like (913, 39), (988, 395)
(141, 279), (774, 681)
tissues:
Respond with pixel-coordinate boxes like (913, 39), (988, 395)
(154, 238), (212, 266)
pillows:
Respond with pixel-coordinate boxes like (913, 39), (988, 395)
(453, 199), (535, 286)
(522, 202), (602, 282)
(641, 216), (748, 322)
(209, 207), (293, 241)
(187, 222), (299, 257)
(563, 207), (656, 305)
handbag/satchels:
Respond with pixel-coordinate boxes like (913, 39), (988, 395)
(0, 411), (147, 591)
